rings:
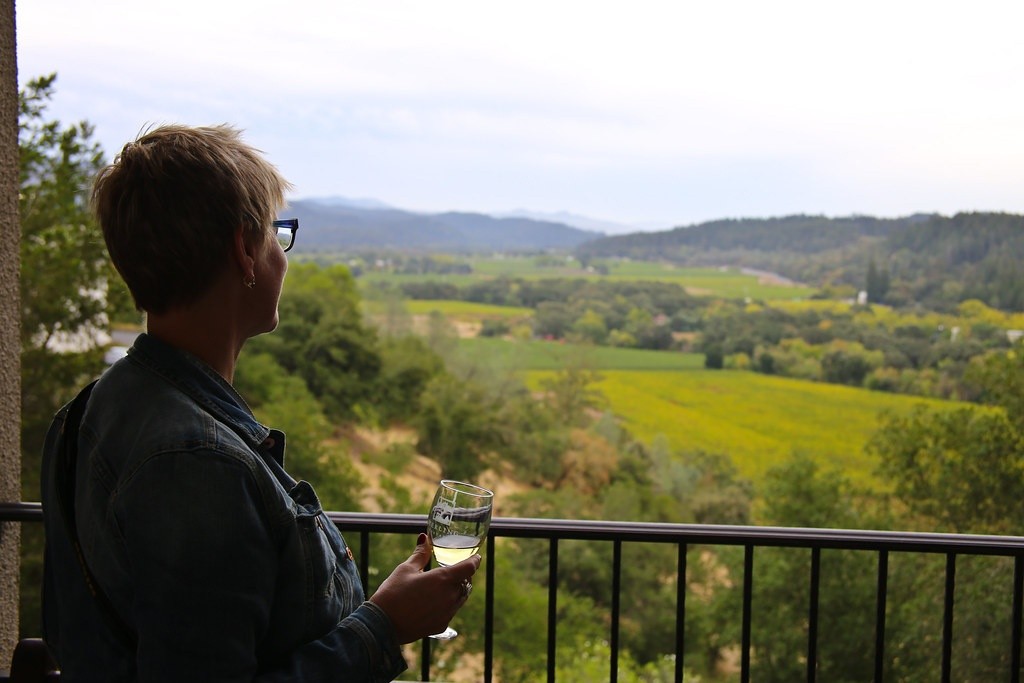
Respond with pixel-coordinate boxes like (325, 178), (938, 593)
(461, 580), (473, 598)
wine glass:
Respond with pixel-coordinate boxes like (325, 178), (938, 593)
(423, 481), (493, 640)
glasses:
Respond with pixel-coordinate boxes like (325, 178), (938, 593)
(271, 218), (298, 253)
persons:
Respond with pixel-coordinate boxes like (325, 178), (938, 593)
(38, 124), (481, 683)
